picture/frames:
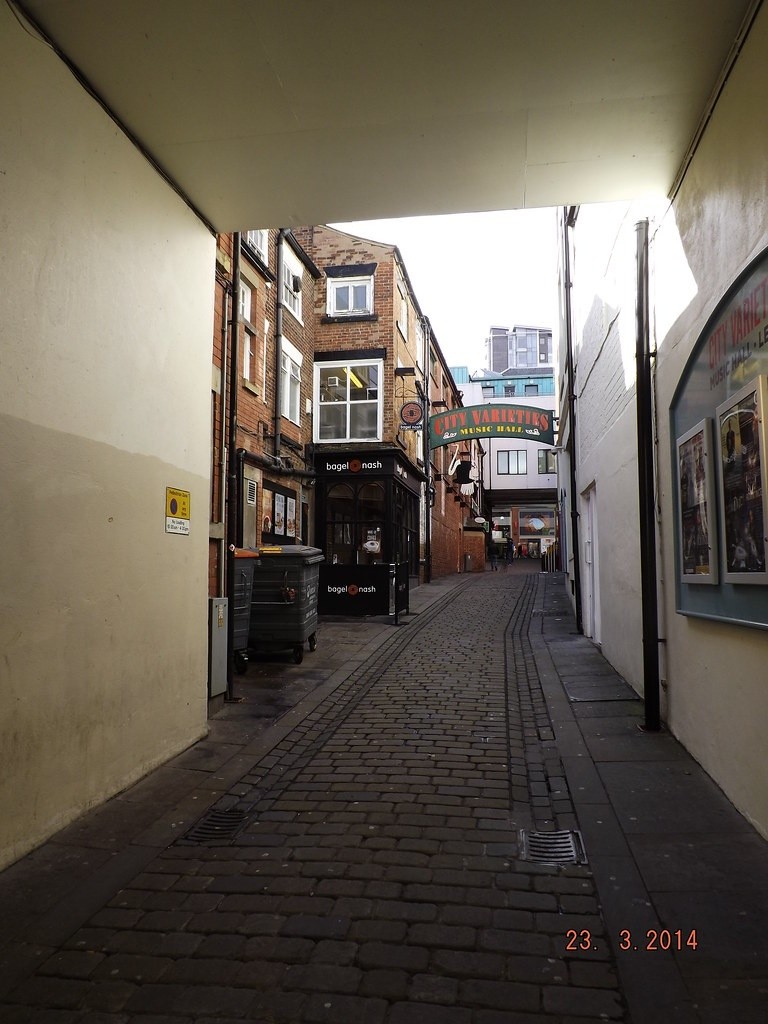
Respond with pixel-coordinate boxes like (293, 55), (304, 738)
(715, 374), (768, 585)
(676, 413), (719, 585)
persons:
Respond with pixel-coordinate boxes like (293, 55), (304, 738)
(487, 539), (499, 571)
(504, 537), (523, 567)
(677, 418), (766, 575)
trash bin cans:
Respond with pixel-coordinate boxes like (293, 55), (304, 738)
(231, 547), (260, 675)
(465, 552), (473, 572)
(248, 545), (326, 665)
